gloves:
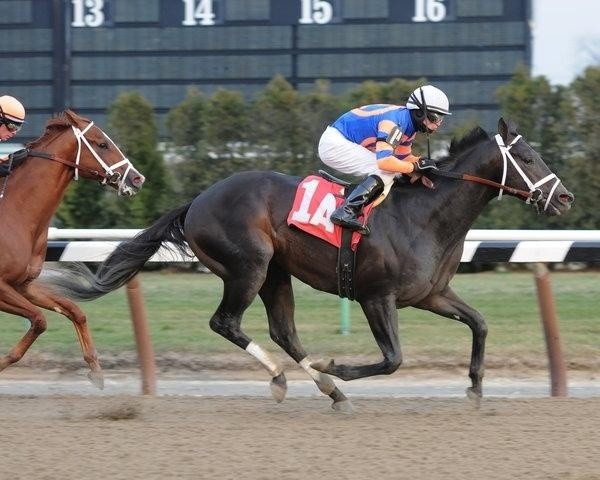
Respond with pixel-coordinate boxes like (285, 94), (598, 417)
(414, 157), (440, 173)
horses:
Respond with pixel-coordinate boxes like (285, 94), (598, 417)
(0, 109), (146, 392)
(39, 116), (574, 414)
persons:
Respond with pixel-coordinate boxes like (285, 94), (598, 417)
(316, 83), (451, 236)
(0, 94), (30, 178)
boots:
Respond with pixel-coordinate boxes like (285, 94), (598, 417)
(329, 176), (386, 237)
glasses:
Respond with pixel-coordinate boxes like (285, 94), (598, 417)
(426, 112), (444, 124)
(5, 121), (22, 133)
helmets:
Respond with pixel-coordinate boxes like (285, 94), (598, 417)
(405, 84), (454, 117)
(0, 95), (26, 123)
